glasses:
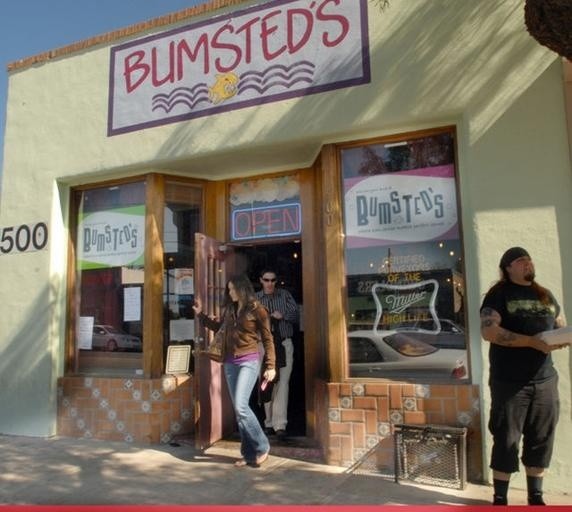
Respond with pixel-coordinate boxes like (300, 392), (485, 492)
(263, 278), (277, 283)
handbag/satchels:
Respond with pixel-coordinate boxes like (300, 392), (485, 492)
(205, 323), (225, 362)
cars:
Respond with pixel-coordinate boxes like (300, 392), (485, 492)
(347, 318), (468, 378)
(91, 323), (142, 351)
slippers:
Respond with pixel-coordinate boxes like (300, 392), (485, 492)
(234, 444), (271, 467)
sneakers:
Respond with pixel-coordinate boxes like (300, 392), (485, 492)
(528, 495), (545, 505)
(493, 496), (507, 505)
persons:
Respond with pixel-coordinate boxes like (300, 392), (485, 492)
(478, 246), (570, 505)
(192, 273), (277, 469)
(250, 268), (300, 439)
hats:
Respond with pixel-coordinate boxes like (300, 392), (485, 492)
(499, 247), (531, 269)
